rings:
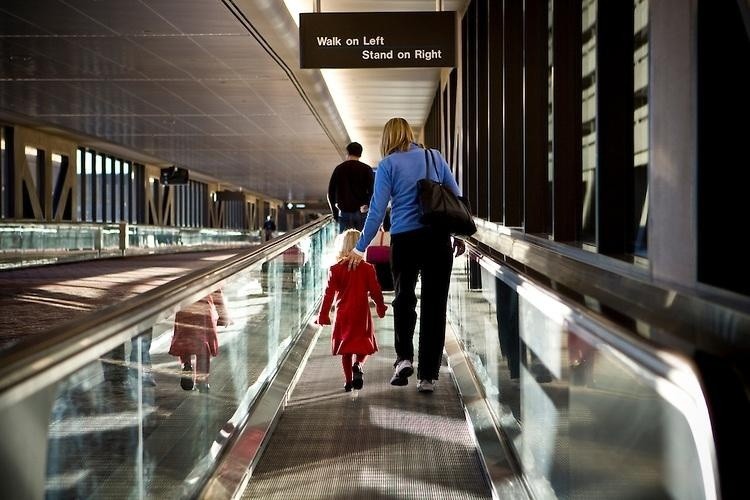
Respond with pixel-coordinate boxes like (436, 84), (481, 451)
(353, 260), (357, 264)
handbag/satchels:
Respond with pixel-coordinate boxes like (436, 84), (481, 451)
(381, 207), (391, 232)
(365, 245), (394, 263)
(417, 179), (478, 237)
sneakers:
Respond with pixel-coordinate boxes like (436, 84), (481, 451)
(112, 379), (133, 394)
(180, 364), (210, 393)
(344, 362), (363, 390)
(391, 359), (413, 387)
(416, 379), (436, 392)
(142, 372), (158, 386)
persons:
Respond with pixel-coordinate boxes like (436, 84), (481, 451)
(313, 230), (388, 392)
(262, 214), (276, 242)
(99, 326), (158, 393)
(339, 116), (476, 390)
(167, 286), (235, 394)
(326, 142), (376, 234)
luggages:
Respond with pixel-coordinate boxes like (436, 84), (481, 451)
(375, 263), (395, 291)
